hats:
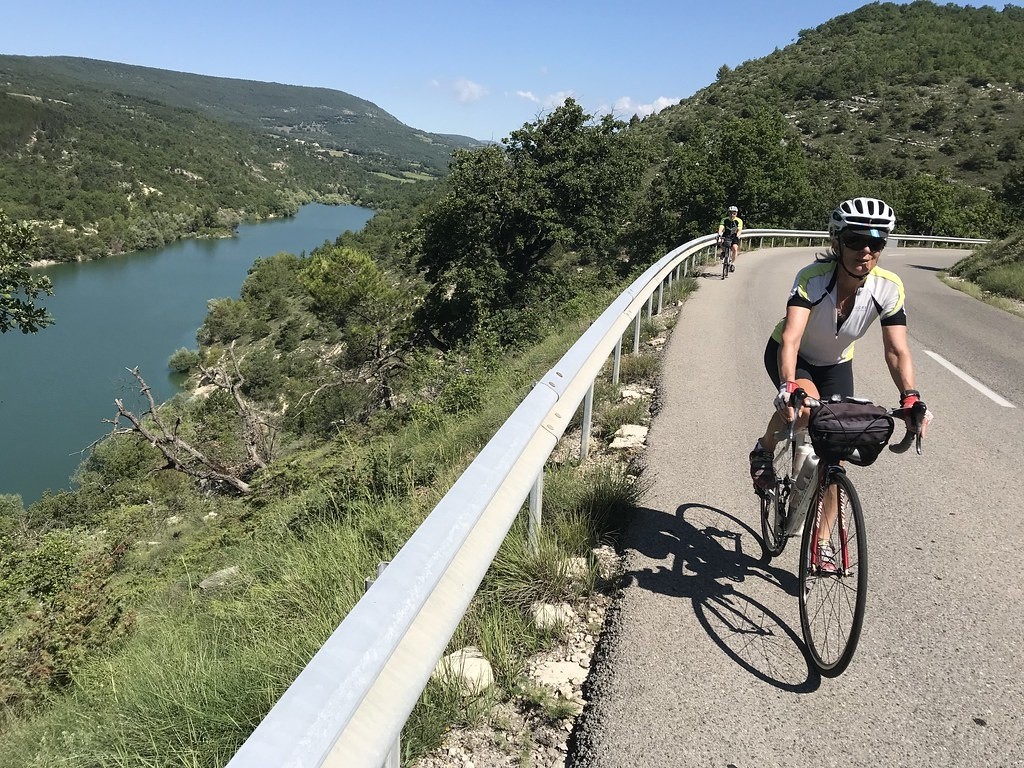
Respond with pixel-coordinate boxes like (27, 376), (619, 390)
(848, 227), (888, 238)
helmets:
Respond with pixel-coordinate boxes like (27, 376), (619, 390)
(728, 206), (738, 213)
(829, 197), (897, 251)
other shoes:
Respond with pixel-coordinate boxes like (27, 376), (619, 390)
(730, 265), (735, 272)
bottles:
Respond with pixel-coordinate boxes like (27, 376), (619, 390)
(792, 442), (814, 471)
(796, 452), (820, 490)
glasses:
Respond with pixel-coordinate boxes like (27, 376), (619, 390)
(842, 235), (888, 254)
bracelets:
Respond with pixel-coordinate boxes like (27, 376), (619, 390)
(899, 389), (920, 408)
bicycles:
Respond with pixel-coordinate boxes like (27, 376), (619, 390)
(719, 235), (733, 280)
(756, 386), (921, 679)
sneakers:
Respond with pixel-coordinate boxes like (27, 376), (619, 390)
(810, 540), (835, 572)
(749, 443), (775, 489)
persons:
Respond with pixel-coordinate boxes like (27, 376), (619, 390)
(717, 206), (743, 272)
(749, 198), (933, 573)
